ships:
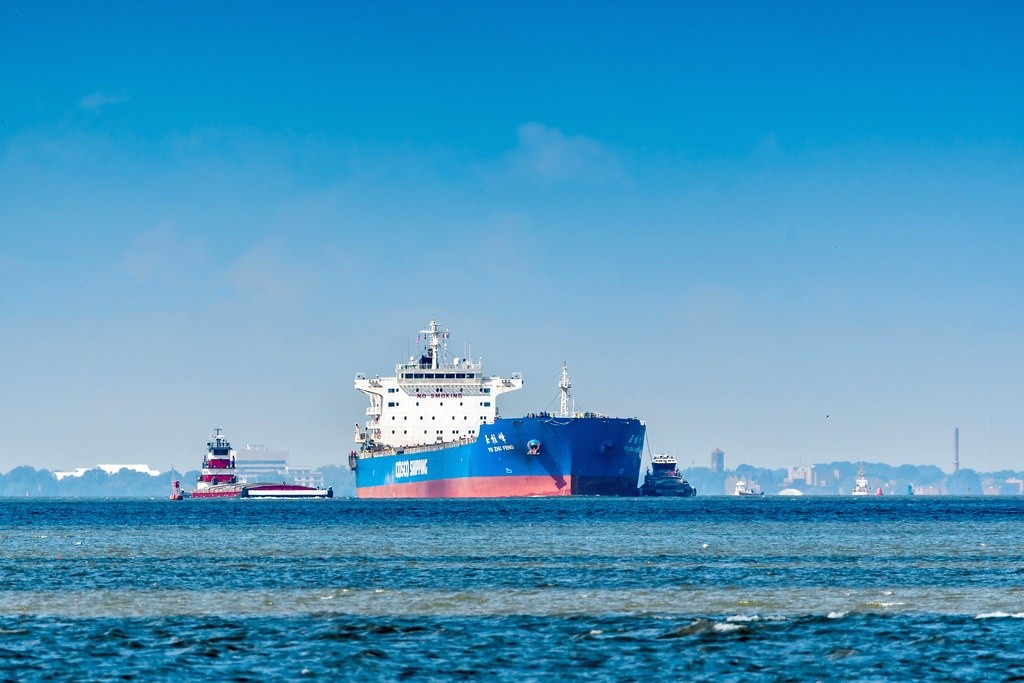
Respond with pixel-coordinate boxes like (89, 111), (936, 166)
(348, 318), (647, 497)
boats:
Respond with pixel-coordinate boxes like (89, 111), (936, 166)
(169, 479), (184, 501)
(192, 427), (334, 498)
(734, 480), (763, 496)
(851, 460), (873, 495)
(638, 435), (697, 496)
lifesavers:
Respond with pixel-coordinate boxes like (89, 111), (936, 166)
(377, 435), (381, 439)
